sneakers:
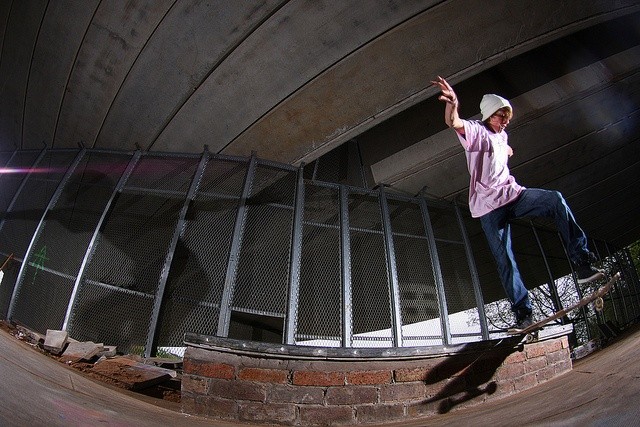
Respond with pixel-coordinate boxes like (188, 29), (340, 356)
(577, 268), (605, 285)
(508, 313), (533, 333)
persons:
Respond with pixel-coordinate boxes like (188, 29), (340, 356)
(430, 75), (606, 329)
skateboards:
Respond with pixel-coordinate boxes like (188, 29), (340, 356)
(508, 272), (621, 343)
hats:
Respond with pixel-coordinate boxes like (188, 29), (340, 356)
(480, 94), (512, 122)
